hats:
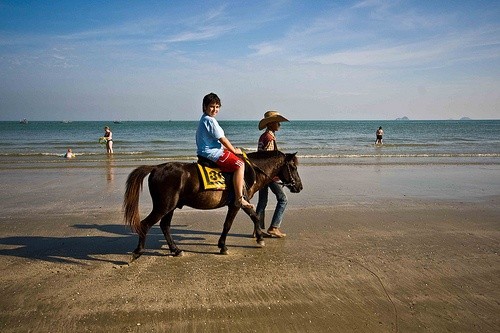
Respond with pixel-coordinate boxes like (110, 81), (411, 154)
(259, 111), (289, 130)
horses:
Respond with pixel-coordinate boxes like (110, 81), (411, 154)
(121, 150), (304, 263)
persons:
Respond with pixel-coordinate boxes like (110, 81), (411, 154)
(65, 149), (73, 158)
(252, 110), (289, 238)
(195, 92), (254, 209)
(100, 126), (113, 153)
(375, 126), (383, 144)
